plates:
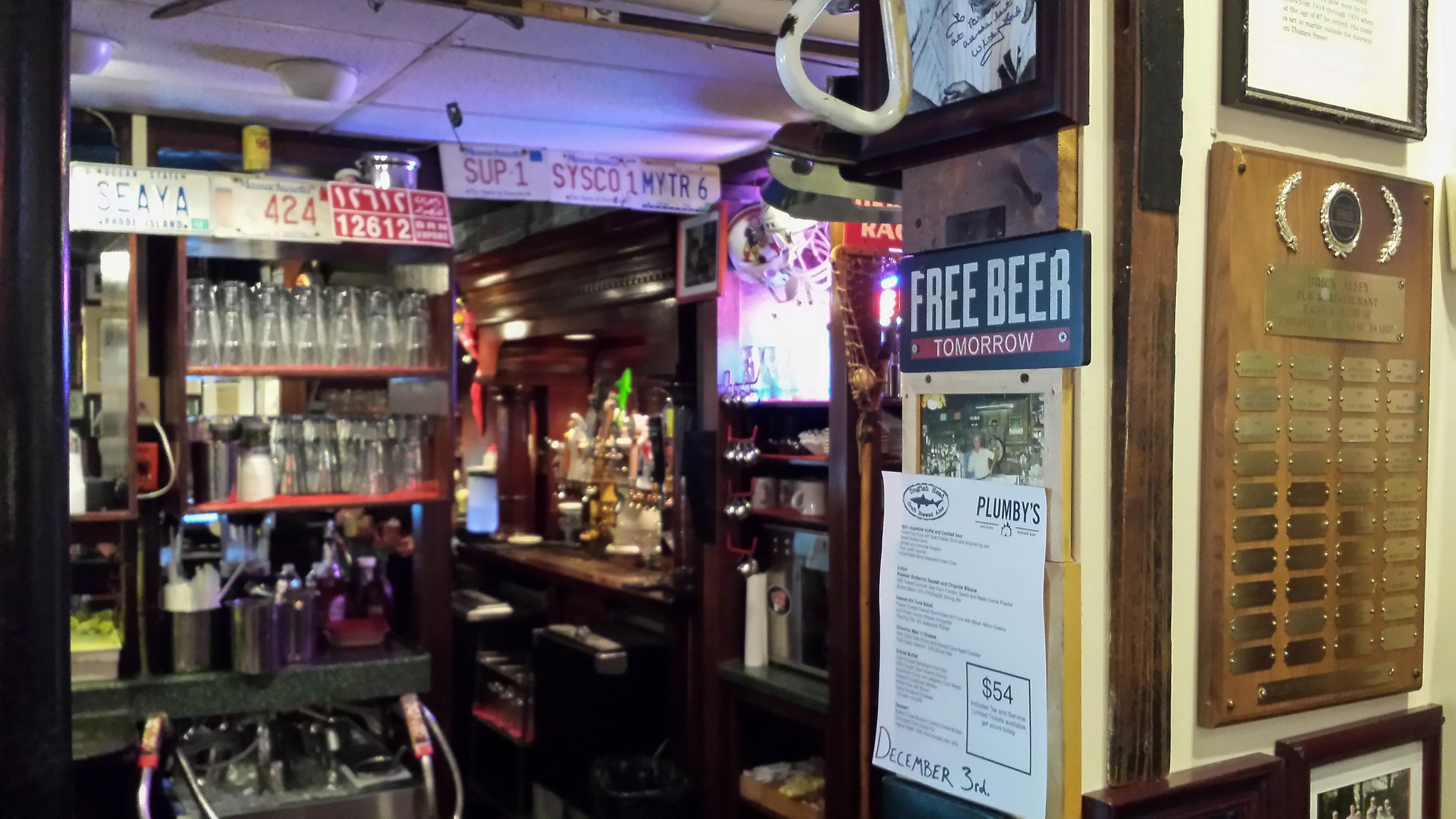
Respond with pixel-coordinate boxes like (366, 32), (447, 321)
(824, 427), (890, 454)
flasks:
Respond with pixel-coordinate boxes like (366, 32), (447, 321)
(187, 413), (230, 504)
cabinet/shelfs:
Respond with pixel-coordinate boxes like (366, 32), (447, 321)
(185, 359), (449, 515)
(735, 453), (830, 535)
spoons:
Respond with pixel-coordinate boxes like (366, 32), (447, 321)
(724, 441), (762, 576)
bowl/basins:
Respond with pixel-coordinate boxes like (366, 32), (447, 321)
(356, 151), (421, 191)
(769, 427), (823, 455)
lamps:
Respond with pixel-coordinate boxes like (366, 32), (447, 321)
(263, 58), (359, 103)
(70, 28), (127, 74)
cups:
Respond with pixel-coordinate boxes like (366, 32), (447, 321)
(750, 478), (830, 516)
(156, 589), (321, 675)
(267, 389), (428, 496)
(186, 275), (430, 367)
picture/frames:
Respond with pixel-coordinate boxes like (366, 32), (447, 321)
(1080, 750), (1287, 819)
(1275, 699), (1443, 819)
(1222, 0), (1431, 141)
(854, 0), (1091, 177)
